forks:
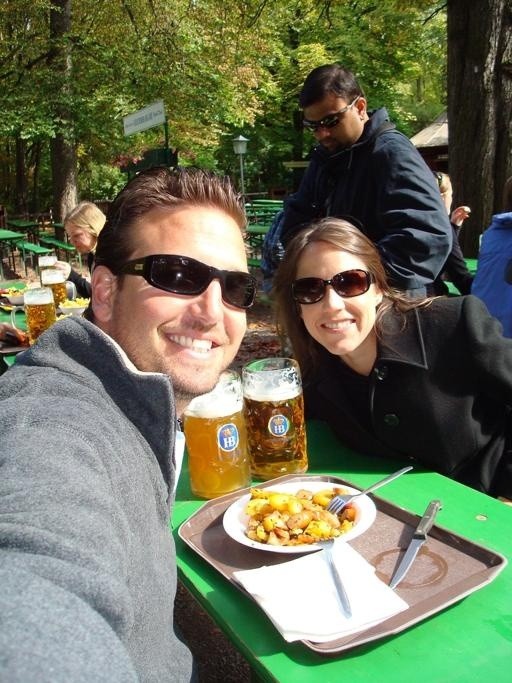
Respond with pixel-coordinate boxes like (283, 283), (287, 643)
(326, 465), (413, 514)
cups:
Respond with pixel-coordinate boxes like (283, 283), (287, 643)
(10, 287), (57, 345)
(243, 356), (309, 480)
(183, 370), (251, 499)
(38, 256), (58, 287)
(42, 270), (77, 307)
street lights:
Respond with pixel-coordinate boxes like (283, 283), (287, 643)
(231, 135), (250, 199)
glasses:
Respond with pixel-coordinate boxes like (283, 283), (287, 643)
(284, 269), (376, 305)
(301, 93), (362, 133)
(119, 254), (258, 310)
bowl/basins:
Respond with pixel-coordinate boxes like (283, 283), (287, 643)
(59, 306), (86, 316)
(7, 295), (24, 304)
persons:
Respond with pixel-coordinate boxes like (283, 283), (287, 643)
(0, 165), (258, 682)
(425, 171), (475, 302)
(279, 62), (454, 303)
(55, 200), (107, 326)
(272, 216), (512, 507)
(260, 209), (287, 300)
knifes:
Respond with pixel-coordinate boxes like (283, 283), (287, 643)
(389, 499), (444, 589)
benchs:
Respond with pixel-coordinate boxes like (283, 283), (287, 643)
(0, 219), (80, 280)
(244, 198), (285, 276)
(444, 280), (461, 295)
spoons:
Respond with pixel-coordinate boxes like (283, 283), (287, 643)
(318, 538), (351, 617)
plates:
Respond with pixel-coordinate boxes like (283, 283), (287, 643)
(224, 482), (376, 553)
